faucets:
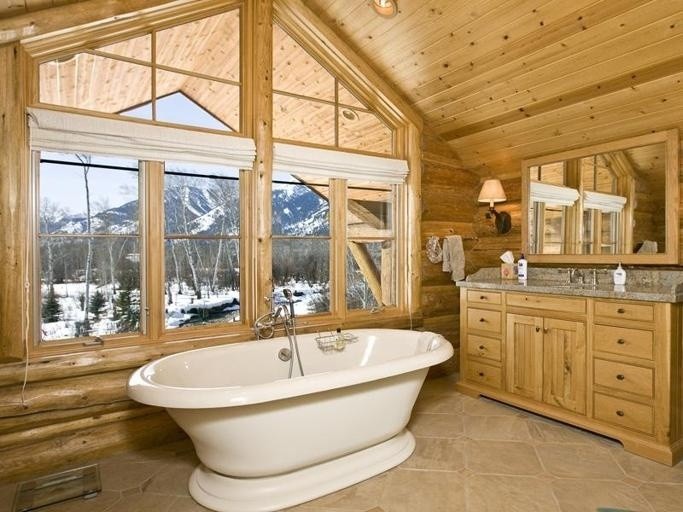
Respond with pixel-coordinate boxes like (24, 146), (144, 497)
(569, 267), (584, 283)
(254, 305), (291, 339)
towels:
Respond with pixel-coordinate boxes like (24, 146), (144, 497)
(442, 235), (465, 282)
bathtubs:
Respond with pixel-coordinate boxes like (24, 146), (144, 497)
(126, 328), (454, 512)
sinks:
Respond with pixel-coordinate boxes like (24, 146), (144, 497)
(553, 284), (600, 290)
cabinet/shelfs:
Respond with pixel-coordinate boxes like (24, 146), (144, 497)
(454, 288), (683, 466)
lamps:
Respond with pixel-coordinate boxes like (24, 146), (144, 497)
(477, 180), (511, 234)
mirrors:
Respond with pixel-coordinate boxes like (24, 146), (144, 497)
(519, 127), (679, 266)
